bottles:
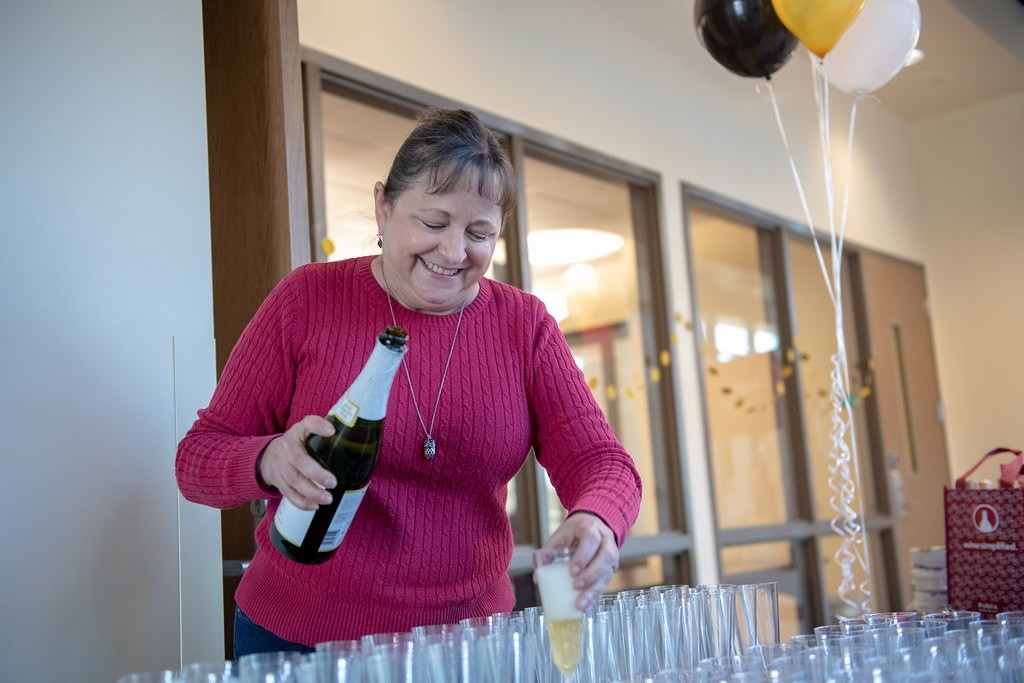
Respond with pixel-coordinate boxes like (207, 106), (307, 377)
(270, 327), (409, 565)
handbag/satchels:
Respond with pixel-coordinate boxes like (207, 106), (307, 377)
(944, 447), (1024, 624)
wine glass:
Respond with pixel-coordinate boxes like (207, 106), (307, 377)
(115, 547), (1024, 683)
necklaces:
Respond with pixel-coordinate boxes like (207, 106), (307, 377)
(382, 263), (466, 462)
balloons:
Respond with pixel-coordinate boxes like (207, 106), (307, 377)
(695, 0), (921, 98)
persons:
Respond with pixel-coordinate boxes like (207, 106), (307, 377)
(174, 109), (642, 661)
